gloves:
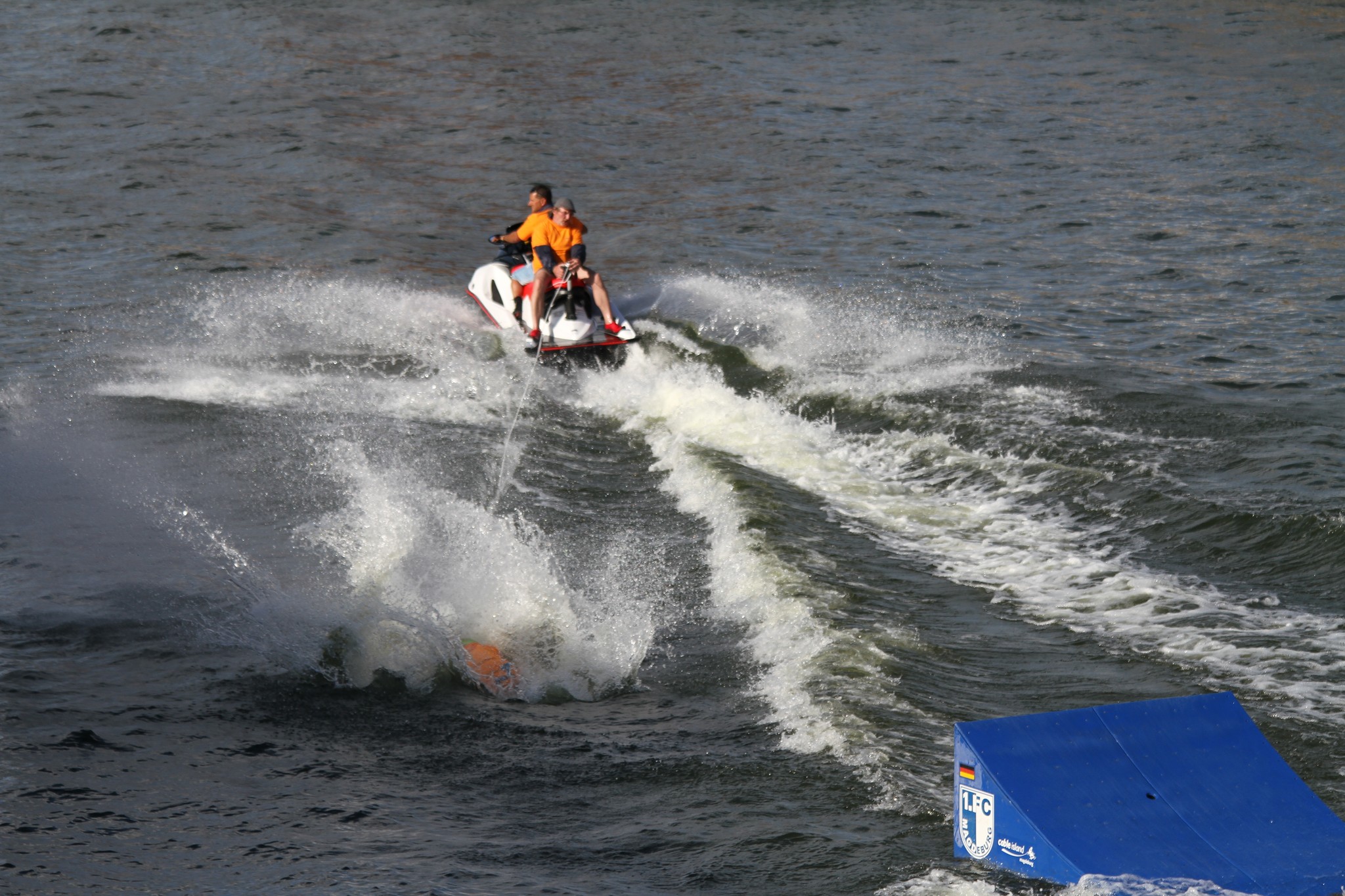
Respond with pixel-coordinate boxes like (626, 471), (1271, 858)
(489, 234), (503, 244)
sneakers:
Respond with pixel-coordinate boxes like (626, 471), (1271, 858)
(605, 322), (622, 334)
(514, 310), (522, 321)
(528, 329), (541, 341)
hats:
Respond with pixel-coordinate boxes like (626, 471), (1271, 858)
(554, 197), (575, 212)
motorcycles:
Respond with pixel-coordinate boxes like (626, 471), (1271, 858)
(465, 220), (637, 353)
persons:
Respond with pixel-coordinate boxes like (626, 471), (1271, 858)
(492, 236), (501, 243)
(500, 184), (588, 321)
(524, 197), (633, 350)
